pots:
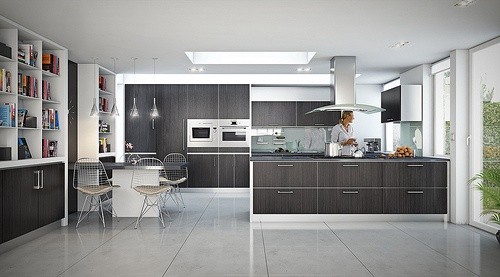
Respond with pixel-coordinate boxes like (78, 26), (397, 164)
(324, 140), (342, 157)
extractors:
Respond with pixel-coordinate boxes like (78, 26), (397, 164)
(304, 57), (386, 115)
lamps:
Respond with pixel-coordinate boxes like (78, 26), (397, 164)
(129, 57), (140, 116)
(89, 57), (102, 117)
(109, 57), (120, 117)
(148, 57), (160, 117)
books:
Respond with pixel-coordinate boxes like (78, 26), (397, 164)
(42, 80), (51, 100)
(18, 43), (36, 67)
(99, 75), (111, 153)
(42, 54), (59, 75)
(0, 102), (16, 127)
(42, 109), (59, 130)
(18, 72), (38, 98)
(0, 66), (12, 94)
(42, 138), (58, 158)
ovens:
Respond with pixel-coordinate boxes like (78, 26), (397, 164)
(219, 119), (250, 147)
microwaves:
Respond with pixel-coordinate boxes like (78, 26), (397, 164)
(187, 119), (220, 146)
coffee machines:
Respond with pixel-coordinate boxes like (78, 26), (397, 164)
(364, 138), (381, 153)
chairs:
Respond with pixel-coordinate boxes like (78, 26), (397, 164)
(72, 157), (120, 229)
(130, 158), (173, 230)
(159, 152), (188, 213)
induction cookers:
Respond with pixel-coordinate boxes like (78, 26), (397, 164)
(320, 154), (377, 158)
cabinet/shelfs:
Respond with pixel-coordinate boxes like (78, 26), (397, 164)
(252, 101), (341, 128)
(380, 84), (422, 124)
(187, 84), (249, 189)
(0, 163), (65, 245)
(250, 161), (449, 223)
(0, 13), (69, 169)
(125, 84), (187, 189)
(77, 63), (116, 212)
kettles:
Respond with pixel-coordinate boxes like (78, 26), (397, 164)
(351, 147), (366, 158)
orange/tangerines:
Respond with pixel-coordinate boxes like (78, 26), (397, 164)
(388, 146), (413, 158)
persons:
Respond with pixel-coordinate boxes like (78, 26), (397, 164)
(331, 111), (356, 155)
